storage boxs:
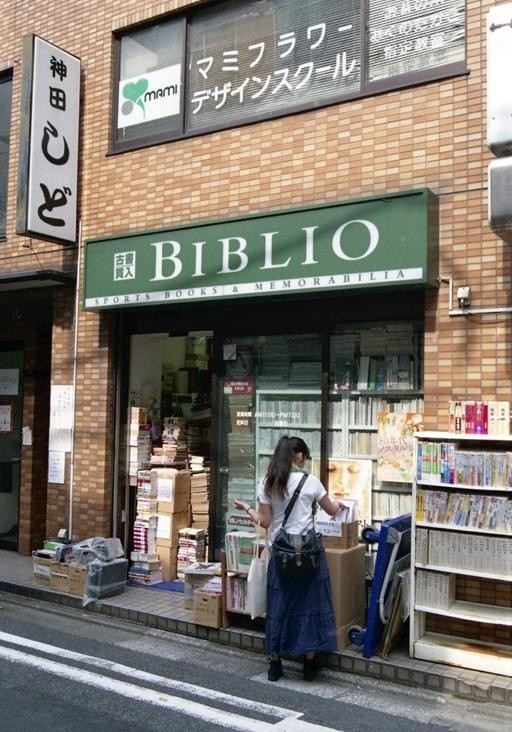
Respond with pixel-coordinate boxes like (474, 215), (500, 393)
(119, 406), (223, 629)
(32, 555), (90, 598)
(320, 520), (367, 652)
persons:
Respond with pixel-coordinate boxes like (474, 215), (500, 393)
(231, 434), (348, 682)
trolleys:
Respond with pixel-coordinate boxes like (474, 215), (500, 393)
(348, 512), (412, 659)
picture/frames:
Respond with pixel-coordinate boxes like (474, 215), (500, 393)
(311, 457), (373, 540)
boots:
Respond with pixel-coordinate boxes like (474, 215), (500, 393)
(302, 659), (317, 681)
(268, 660), (283, 681)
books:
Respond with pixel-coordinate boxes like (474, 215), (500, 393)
(422, 440), (512, 611)
(153, 415), (201, 462)
(223, 323), (423, 610)
(128, 436), (151, 486)
(176, 528), (222, 593)
(449, 400), (510, 434)
(129, 470), (163, 585)
(190, 454), (214, 563)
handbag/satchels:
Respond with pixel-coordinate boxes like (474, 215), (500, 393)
(271, 529), (319, 576)
(246, 548), (270, 621)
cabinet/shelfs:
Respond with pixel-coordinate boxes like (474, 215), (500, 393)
(346, 389), (425, 552)
(409, 431), (512, 677)
(256, 389), (345, 538)
